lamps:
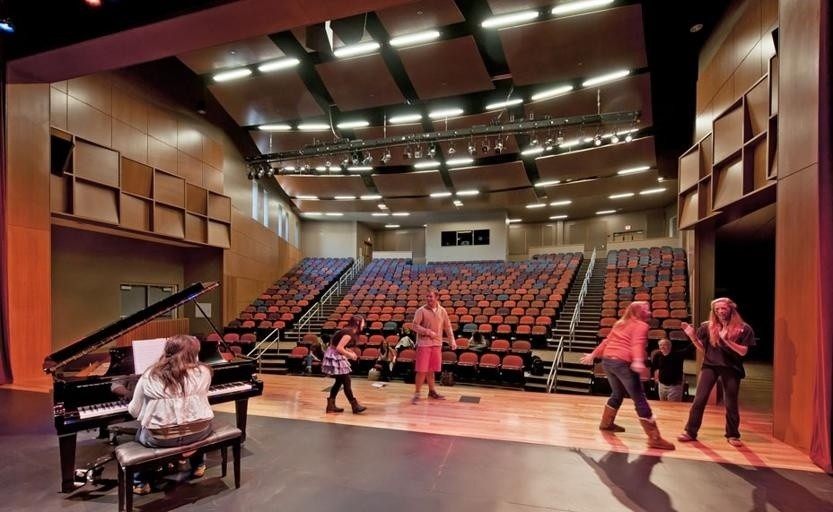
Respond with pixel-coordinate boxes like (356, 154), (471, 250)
(278, 160), (284, 173)
(339, 153), (349, 169)
(528, 127), (586, 150)
(247, 164), (255, 180)
(480, 134), (490, 154)
(360, 150), (373, 167)
(403, 144), (411, 159)
(414, 143), (422, 158)
(466, 136), (476, 156)
(426, 142), (436, 159)
(625, 131), (632, 143)
(255, 163), (264, 179)
(324, 154), (331, 167)
(592, 125), (602, 146)
(303, 157), (310, 174)
(379, 147), (391, 165)
(351, 151), (358, 166)
(611, 124), (620, 144)
(447, 139), (456, 158)
(267, 162), (273, 178)
(294, 159), (300, 173)
(493, 133), (504, 154)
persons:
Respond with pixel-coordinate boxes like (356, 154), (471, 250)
(110, 375), (141, 402)
(321, 313), (367, 415)
(650, 339), (683, 402)
(676, 297), (756, 448)
(302, 341), (324, 374)
(579, 301), (675, 451)
(410, 284), (455, 407)
(394, 320), (416, 352)
(468, 331), (487, 355)
(127, 335), (215, 495)
(376, 340), (396, 382)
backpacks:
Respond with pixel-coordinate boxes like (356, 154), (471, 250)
(529, 356), (543, 376)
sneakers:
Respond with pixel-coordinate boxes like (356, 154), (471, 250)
(727, 437), (743, 448)
(412, 392), (421, 405)
(429, 390), (446, 399)
(133, 484), (150, 496)
(192, 466), (207, 478)
(677, 433), (692, 442)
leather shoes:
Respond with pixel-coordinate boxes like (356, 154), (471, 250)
(307, 369), (312, 373)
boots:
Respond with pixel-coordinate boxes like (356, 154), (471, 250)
(638, 416), (676, 450)
(350, 398), (367, 414)
(326, 398), (345, 413)
(599, 404), (625, 432)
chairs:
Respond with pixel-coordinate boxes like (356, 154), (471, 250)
(668, 292), (685, 300)
(670, 309), (688, 318)
(499, 355), (525, 376)
(651, 301), (668, 308)
(282, 295), (292, 300)
(669, 330), (688, 341)
(358, 348), (378, 364)
(253, 313), (266, 318)
(404, 315), (414, 321)
(311, 361), (322, 366)
(272, 320), (285, 328)
(603, 246), (686, 293)
(225, 346), (241, 353)
(267, 306), (278, 311)
(327, 313), (341, 321)
(462, 323), (477, 332)
(356, 335), (367, 345)
(540, 308), (555, 316)
(401, 322), (412, 333)
(454, 307), (468, 314)
(222, 333), (238, 342)
(525, 307), (540, 315)
(276, 300), (285, 305)
(601, 308), (616, 317)
(369, 307), (381, 314)
(518, 316), (535, 325)
(356, 306), (369, 313)
(290, 306), (301, 313)
(441, 337), (449, 350)
(474, 315), (487, 323)
(286, 300), (296, 305)
(482, 307), (496, 315)
(321, 321), (337, 329)
(456, 352), (478, 373)
(515, 325), (531, 334)
(651, 309), (669, 317)
(280, 313), (293, 319)
(451, 323), (458, 331)
(662, 319), (681, 329)
(261, 256), (353, 294)
(510, 307), (525, 315)
(405, 307), (417, 313)
(597, 328), (612, 337)
(239, 333), (256, 342)
(634, 293), (650, 300)
(228, 320), (240, 327)
(601, 300), (617, 308)
(468, 307), (481, 315)
(448, 314), (459, 322)
(392, 307), (405, 313)
(647, 329), (666, 339)
(339, 252), (583, 307)
(299, 335), (318, 348)
(256, 306), (266, 312)
(303, 294), (314, 300)
(669, 301), (686, 309)
(535, 316), (552, 325)
(511, 340), (531, 353)
(617, 309), (625, 316)
(382, 322), (397, 333)
(488, 315), (503, 324)
(600, 317), (616, 326)
(292, 294), (303, 300)
(651, 293), (667, 300)
(593, 361), (607, 379)
(459, 314), (473, 323)
(297, 300), (308, 306)
(384, 335), (399, 346)
(244, 306), (255, 312)
(264, 300), (274, 305)
(267, 312), (279, 318)
(496, 324), (511, 333)
(241, 321), (255, 327)
(602, 293), (617, 300)
(496, 307), (510, 315)
(441, 351), (457, 366)
(391, 314), (404, 321)
(345, 307), (357, 313)
(287, 346), (308, 365)
(647, 318), (660, 329)
(260, 295), (271, 299)
(378, 314), (391, 321)
(380, 307), (393, 313)
(618, 301), (631, 308)
(367, 335), (384, 346)
(490, 339), (509, 352)
(334, 307), (346, 313)
(340, 313), (353, 321)
(257, 320), (271, 328)
(335, 321), (347, 329)
(253, 300), (263, 305)
(240, 313), (252, 318)
(478, 324), (492, 332)
(206, 333), (221, 341)
(396, 350), (416, 371)
(477, 353), (501, 374)
(531, 325), (547, 335)
(271, 295), (282, 299)
(445, 307), (454, 314)
(503, 316), (519, 324)
(455, 337), (468, 349)
(365, 314), (379, 320)
(368, 321), (382, 333)
(618, 293), (633, 300)
(349, 347), (361, 361)
(279, 306), (289, 311)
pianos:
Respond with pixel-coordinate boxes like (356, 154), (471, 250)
(41, 281), (264, 495)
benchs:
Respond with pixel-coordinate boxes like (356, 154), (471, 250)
(114, 419), (241, 512)
